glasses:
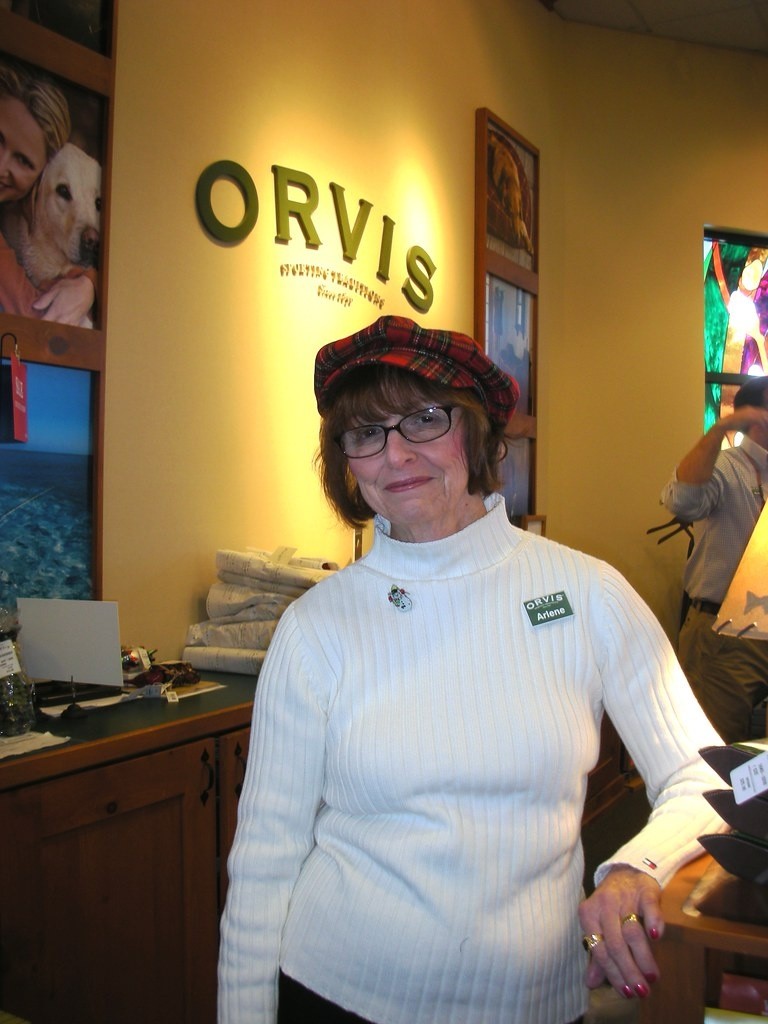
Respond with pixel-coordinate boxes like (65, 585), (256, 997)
(332, 402), (459, 459)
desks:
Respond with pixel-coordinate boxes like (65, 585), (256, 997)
(640, 852), (768, 1024)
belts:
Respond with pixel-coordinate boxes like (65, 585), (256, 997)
(692, 601), (722, 616)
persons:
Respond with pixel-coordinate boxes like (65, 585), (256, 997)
(217, 315), (742, 1024)
(0, 57), (98, 329)
(659, 378), (768, 745)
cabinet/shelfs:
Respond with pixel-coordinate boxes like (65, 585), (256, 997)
(0, 667), (257, 1023)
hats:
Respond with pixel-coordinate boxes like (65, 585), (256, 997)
(313, 318), (520, 432)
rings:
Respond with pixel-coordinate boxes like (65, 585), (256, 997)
(621, 913), (643, 926)
(582, 933), (604, 952)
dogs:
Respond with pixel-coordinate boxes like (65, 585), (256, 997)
(1, 142), (103, 331)
(487, 131), (534, 257)
